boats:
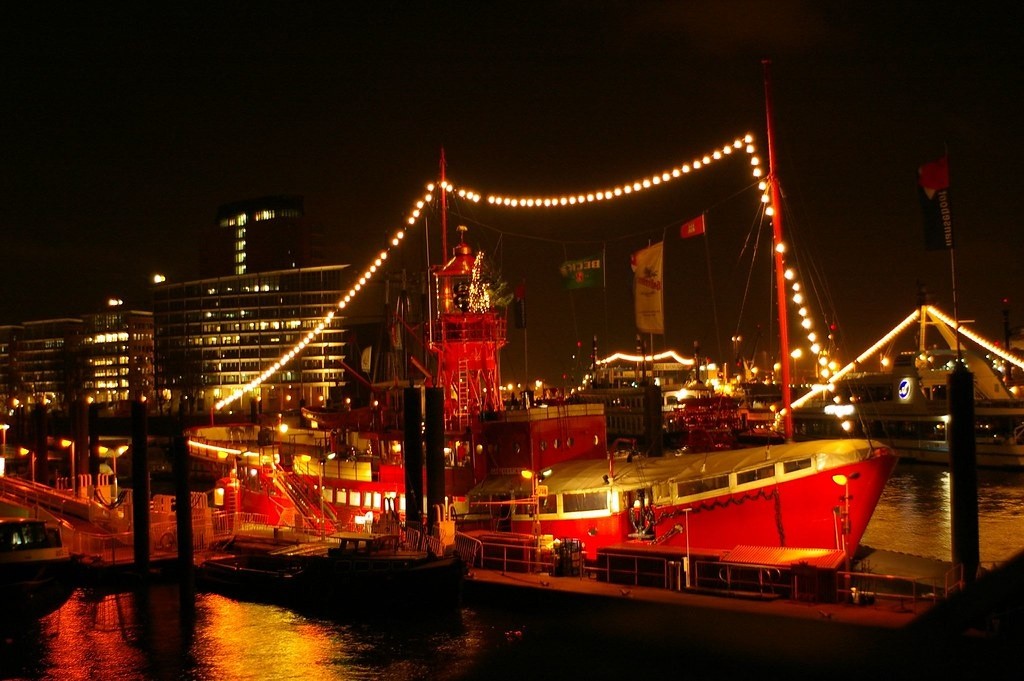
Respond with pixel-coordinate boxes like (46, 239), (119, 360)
(0, 57), (1024, 633)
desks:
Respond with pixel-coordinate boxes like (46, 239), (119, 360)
(330, 531), (402, 557)
